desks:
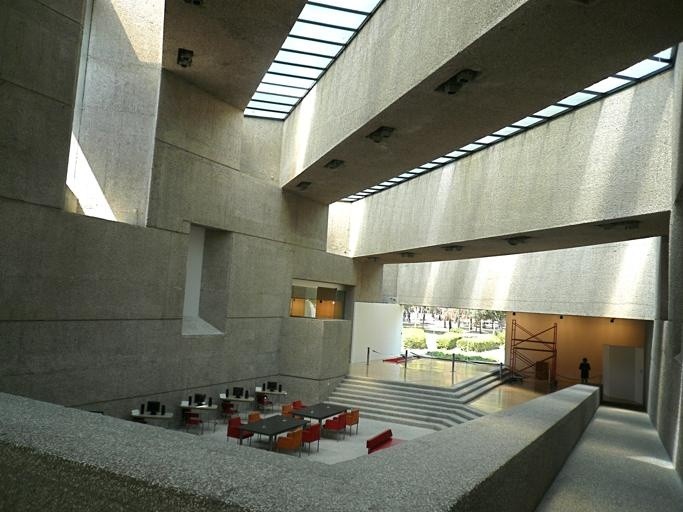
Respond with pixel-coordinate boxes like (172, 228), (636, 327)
(130, 400), (218, 434)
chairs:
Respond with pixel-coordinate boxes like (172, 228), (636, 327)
(219, 386), (360, 458)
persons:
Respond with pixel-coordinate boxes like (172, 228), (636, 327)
(578, 357), (590, 384)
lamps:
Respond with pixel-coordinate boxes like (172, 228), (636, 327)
(432, 67), (480, 96)
(364, 125), (396, 144)
(176, 47), (194, 68)
